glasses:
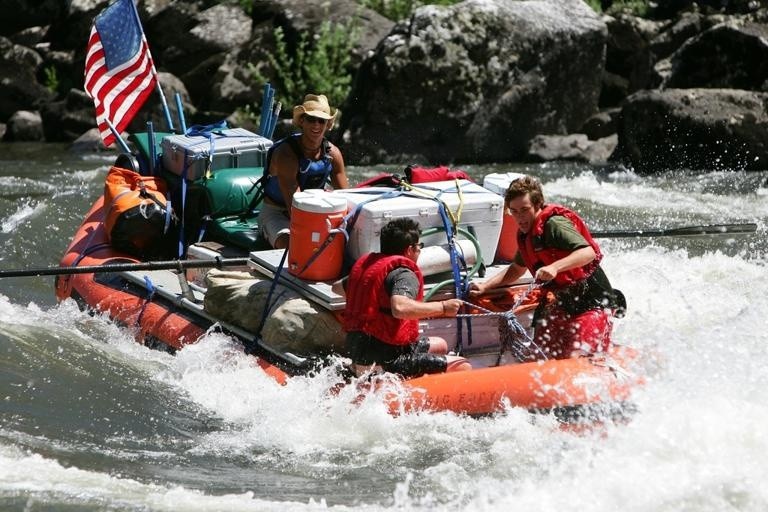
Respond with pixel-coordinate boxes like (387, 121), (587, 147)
(511, 205), (533, 216)
(306, 116), (327, 124)
(415, 243), (424, 249)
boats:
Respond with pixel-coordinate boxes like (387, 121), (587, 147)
(50, 134), (646, 437)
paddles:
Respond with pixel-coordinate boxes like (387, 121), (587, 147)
(589, 223), (757, 238)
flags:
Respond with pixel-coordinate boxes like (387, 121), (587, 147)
(83, 0), (160, 150)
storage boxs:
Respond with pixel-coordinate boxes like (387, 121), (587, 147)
(159, 128), (274, 182)
(333, 179), (505, 267)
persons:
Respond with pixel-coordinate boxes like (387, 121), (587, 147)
(256, 89), (348, 247)
(329, 213), (472, 379)
(465, 172), (628, 363)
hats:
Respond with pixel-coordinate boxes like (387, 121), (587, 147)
(293, 94), (338, 129)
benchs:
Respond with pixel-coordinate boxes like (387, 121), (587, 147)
(127, 132), (179, 179)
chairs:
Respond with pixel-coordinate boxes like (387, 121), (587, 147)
(196, 167), (272, 259)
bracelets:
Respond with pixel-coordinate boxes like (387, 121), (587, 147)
(441, 300), (447, 314)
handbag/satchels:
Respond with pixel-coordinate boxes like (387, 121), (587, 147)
(104, 166), (176, 253)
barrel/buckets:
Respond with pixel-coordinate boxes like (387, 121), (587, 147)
(289, 188), (348, 279)
(483, 172), (519, 263)
